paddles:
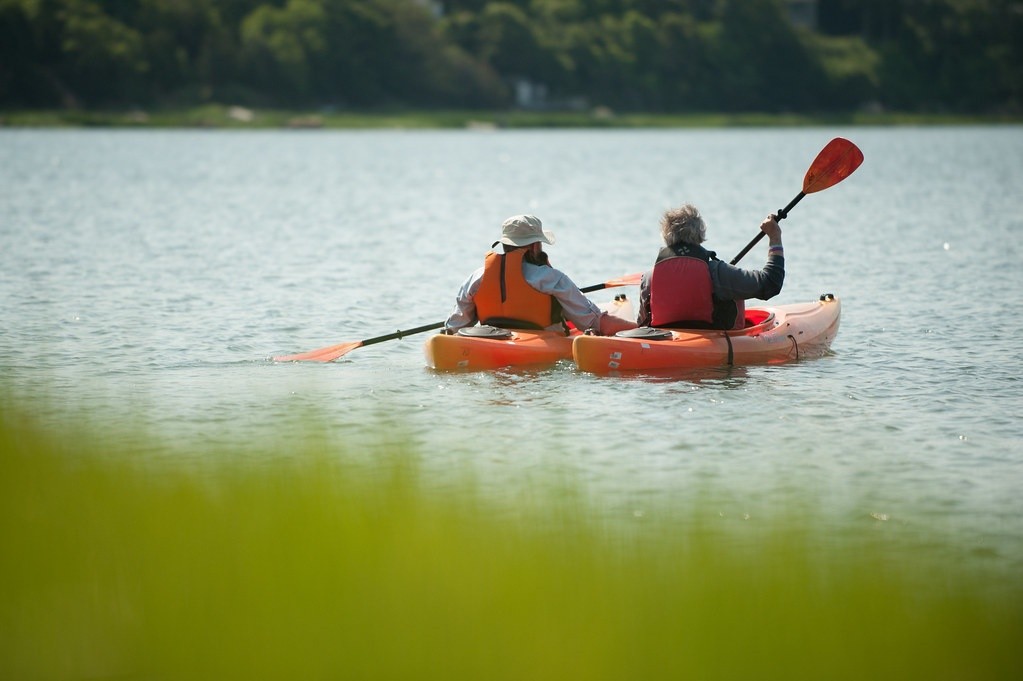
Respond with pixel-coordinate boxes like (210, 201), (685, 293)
(270, 270), (643, 364)
(728, 136), (864, 265)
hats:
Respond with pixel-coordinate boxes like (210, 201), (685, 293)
(492, 215), (556, 249)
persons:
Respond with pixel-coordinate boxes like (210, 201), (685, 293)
(444, 214), (637, 336)
(637, 203), (786, 329)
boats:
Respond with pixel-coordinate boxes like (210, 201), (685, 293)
(425, 292), (841, 377)
